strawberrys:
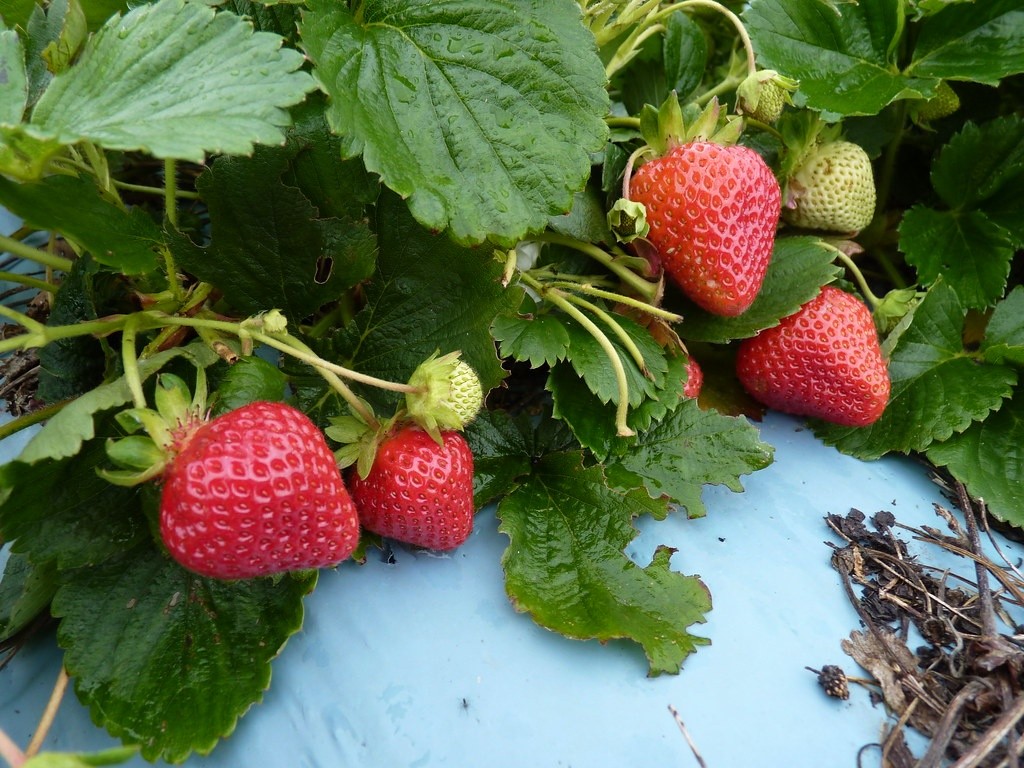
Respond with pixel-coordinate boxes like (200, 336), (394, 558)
(155, 68), (894, 582)
